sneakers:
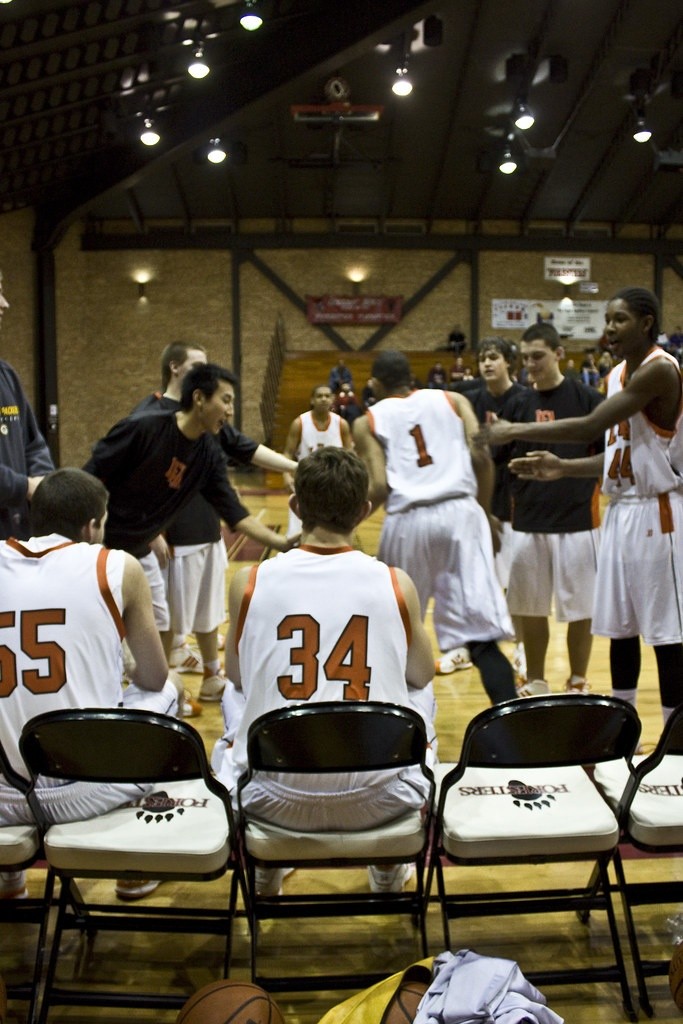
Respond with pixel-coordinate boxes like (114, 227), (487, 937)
(435, 646), (473, 674)
(257, 868), (294, 896)
(516, 681), (552, 698)
(199, 677), (228, 701)
(367, 862), (412, 892)
(181, 693), (201, 716)
(563, 680), (591, 694)
(115, 877), (162, 896)
(168, 646), (222, 673)
(0, 871), (30, 899)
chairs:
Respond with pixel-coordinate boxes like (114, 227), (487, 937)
(0, 694), (683, 1024)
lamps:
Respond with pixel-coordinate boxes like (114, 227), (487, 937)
(499, 142), (517, 174)
(634, 103), (652, 143)
(392, 56), (413, 96)
(352, 282), (362, 296)
(512, 97), (535, 130)
(138, 282), (145, 300)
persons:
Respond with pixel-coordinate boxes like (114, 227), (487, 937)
(352, 351), (519, 707)
(283, 385), (351, 546)
(0, 269), (185, 898)
(82, 340), (301, 717)
(329, 359), (423, 434)
(211, 446), (438, 895)
(428, 288), (683, 755)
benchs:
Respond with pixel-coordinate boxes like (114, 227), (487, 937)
(269, 349), (616, 490)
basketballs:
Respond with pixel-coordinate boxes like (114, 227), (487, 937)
(176, 978), (286, 1024)
(381, 981), (430, 1024)
